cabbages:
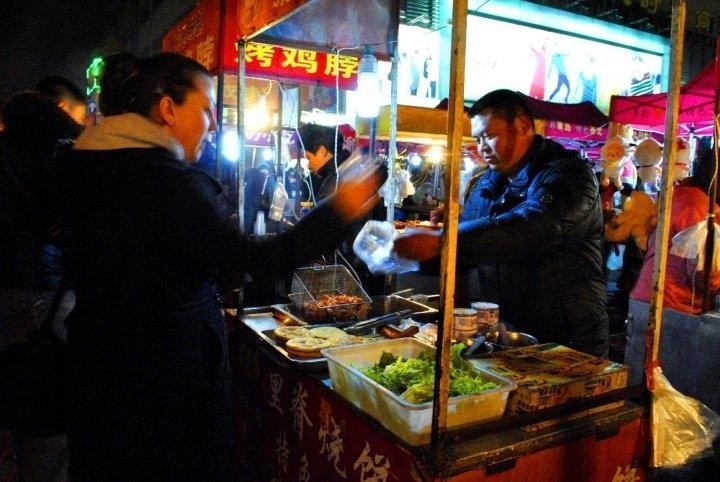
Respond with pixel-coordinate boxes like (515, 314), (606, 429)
(342, 340), (504, 402)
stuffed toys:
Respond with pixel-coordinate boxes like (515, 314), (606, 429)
(602, 136), (629, 190)
(604, 191), (661, 252)
(674, 136), (692, 184)
(605, 121), (634, 144)
(634, 138), (664, 185)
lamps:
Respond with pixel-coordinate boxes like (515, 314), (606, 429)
(357, 44), (379, 117)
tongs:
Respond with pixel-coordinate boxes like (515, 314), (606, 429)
(345, 309), (412, 336)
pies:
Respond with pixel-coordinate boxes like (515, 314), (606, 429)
(273, 325), (366, 358)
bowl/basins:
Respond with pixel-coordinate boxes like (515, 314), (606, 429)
(320, 337), (510, 446)
(485, 331), (538, 348)
(450, 338), (493, 358)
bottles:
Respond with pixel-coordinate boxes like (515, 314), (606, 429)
(470, 301), (499, 344)
(452, 307), (479, 345)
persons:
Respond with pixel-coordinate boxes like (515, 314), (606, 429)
(29, 50), (382, 482)
(0, 75), (88, 482)
(305, 126), (351, 204)
(190, 139), (237, 201)
(398, 45), (437, 99)
(619, 55), (661, 97)
(572, 54), (600, 105)
(243, 160), (305, 236)
(624, 149), (720, 386)
(393, 88), (611, 360)
(548, 44), (573, 104)
(529, 45), (547, 101)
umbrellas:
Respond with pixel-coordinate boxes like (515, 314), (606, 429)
(435, 98), (470, 113)
(517, 91), (609, 129)
(608, 56), (719, 137)
